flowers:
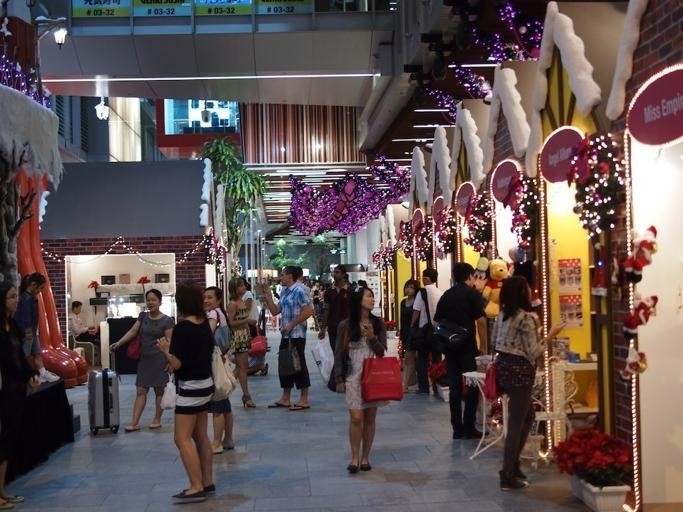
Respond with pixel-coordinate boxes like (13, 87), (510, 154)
(385, 321), (397, 330)
(428, 361), (450, 386)
(550, 425), (629, 489)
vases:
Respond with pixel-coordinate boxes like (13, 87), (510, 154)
(436, 383), (450, 402)
(572, 473), (632, 512)
(386, 331), (396, 338)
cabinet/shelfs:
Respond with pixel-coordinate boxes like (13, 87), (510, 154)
(567, 362), (599, 414)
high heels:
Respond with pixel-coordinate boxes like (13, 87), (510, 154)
(241, 395), (255, 408)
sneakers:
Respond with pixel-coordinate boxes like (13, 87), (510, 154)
(452, 426), (489, 440)
(38, 367), (59, 383)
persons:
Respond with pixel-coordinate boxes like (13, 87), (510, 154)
(69, 301), (101, 366)
(334, 284), (388, 472)
(203, 286), (234, 453)
(155, 279), (216, 504)
(14, 274), (60, 382)
(1, 282), (24, 508)
(624, 225), (657, 283)
(400, 280), (421, 342)
(434, 263), (488, 440)
(108, 290), (175, 431)
(493, 276), (567, 491)
(410, 268), (443, 395)
(225, 265), (367, 411)
(623, 295), (657, 335)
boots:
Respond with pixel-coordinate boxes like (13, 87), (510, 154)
(498, 461), (530, 491)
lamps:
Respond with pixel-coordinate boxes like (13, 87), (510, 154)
(52, 20), (68, 50)
(94, 96), (109, 120)
(201, 100), (210, 122)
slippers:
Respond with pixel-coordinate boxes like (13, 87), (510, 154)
(259, 363), (268, 377)
(1, 495), (24, 510)
(288, 403), (310, 411)
(267, 400), (291, 409)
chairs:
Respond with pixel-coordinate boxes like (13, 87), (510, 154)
(73, 335), (95, 366)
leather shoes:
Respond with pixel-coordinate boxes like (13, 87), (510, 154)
(148, 422), (161, 429)
(221, 440), (234, 449)
(210, 443), (223, 454)
(182, 483), (215, 495)
(360, 459), (372, 472)
(346, 461), (359, 473)
(125, 424), (139, 431)
(170, 489), (206, 504)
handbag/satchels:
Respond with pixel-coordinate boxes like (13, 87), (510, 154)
(248, 324), (269, 356)
(207, 323), (231, 357)
(485, 358), (505, 400)
(209, 346), (236, 400)
(126, 312), (144, 359)
(326, 355), (350, 393)
(433, 321), (474, 350)
(277, 330), (300, 377)
(360, 340), (403, 402)
(406, 324), (435, 350)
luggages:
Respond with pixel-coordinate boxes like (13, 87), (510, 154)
(86, 368), (121, 433)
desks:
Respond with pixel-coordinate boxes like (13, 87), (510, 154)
(463, 369), (546, 461)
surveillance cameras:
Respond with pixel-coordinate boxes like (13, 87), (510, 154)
(201, 110), (209, 122)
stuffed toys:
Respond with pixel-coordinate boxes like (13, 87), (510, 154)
(471, 256), (490, 293)
(482, 259), (513, 318)
